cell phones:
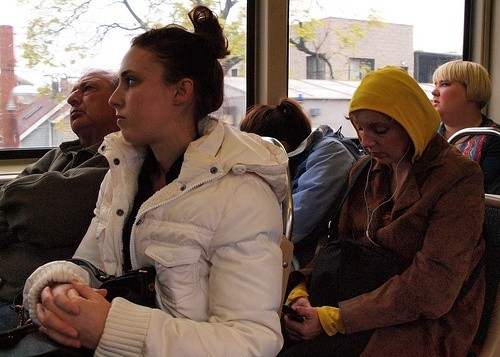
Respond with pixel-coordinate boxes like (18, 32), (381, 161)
(282, 305), (304, 323)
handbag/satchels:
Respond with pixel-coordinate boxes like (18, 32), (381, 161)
(306, 238), (412, 305)
(0, 258), (156, 357)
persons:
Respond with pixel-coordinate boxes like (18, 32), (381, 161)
(429, 61), (499, 195)
(281, 67), (487, 357)
(0, 7), (289, 357)
(241, 99), (364, 245)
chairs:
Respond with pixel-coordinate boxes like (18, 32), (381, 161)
(278, 126), (500, 357)
